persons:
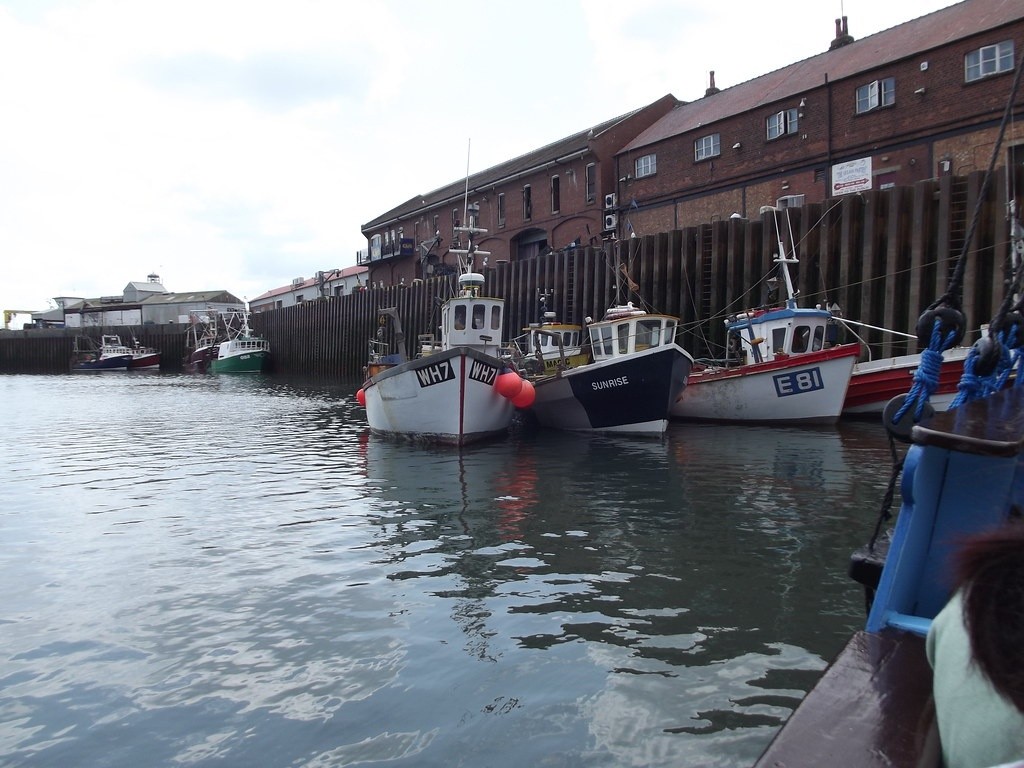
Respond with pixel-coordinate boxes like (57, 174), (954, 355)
(459, 310), (477, 330)
(237, 322), (244, 340)
(924, 527), (1024, 768)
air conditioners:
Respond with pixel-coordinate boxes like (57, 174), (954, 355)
(605, 193), (616, 209)
(605, 214), (616, 229)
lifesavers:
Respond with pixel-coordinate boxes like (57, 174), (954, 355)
(723, 312), (754, 324)
(605, 310), (647, 319)
(605, 307), (640, 312)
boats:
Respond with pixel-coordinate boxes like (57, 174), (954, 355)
(66, 331), (161, 376)
(357, 134), (538, 448)
(672, 208), (863, 427)
(512, 206), (698, 442)
(753, 54), (1022, 768)
(840, 343), (1024, 423)
(180, 309), (270, 373)
